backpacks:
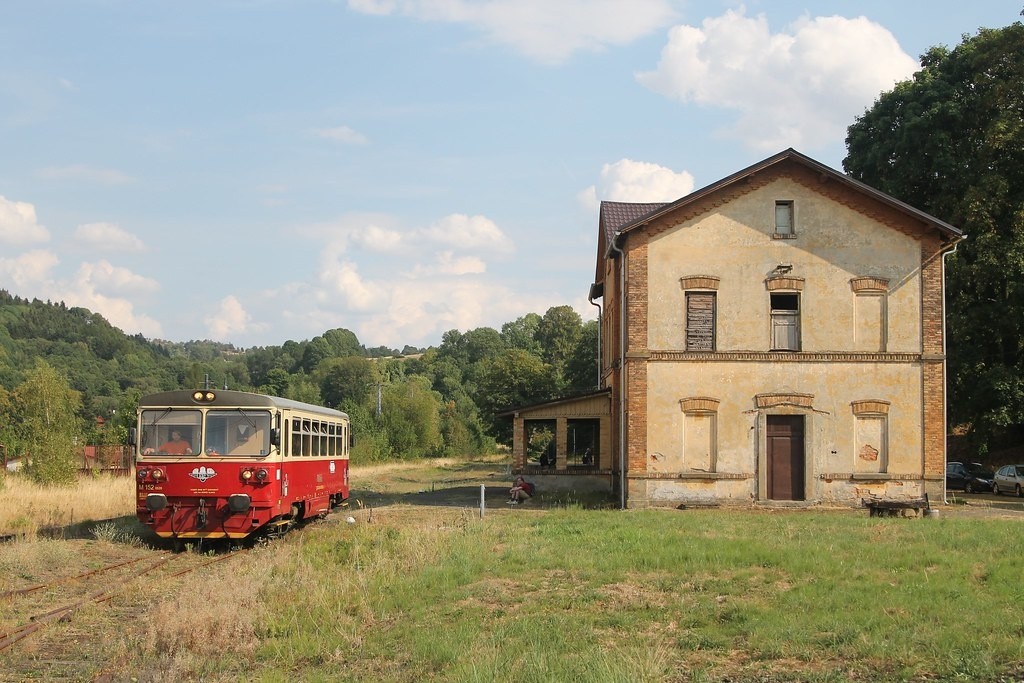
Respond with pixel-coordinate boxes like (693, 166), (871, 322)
(526, 483), (536, 496)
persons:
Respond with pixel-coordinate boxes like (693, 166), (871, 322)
(582, 448), (592, 464)
(506, 476), (532, 505)
(144, 429), (192, 454)
(540, 453), (549, 467)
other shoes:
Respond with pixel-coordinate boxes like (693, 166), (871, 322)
(506, 499), (519, 505)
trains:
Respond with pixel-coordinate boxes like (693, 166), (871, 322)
(126, 384), (355, 548)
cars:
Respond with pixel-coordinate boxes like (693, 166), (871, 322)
(946, 460), (995, 494)
(991, 464), (1024, 497)
